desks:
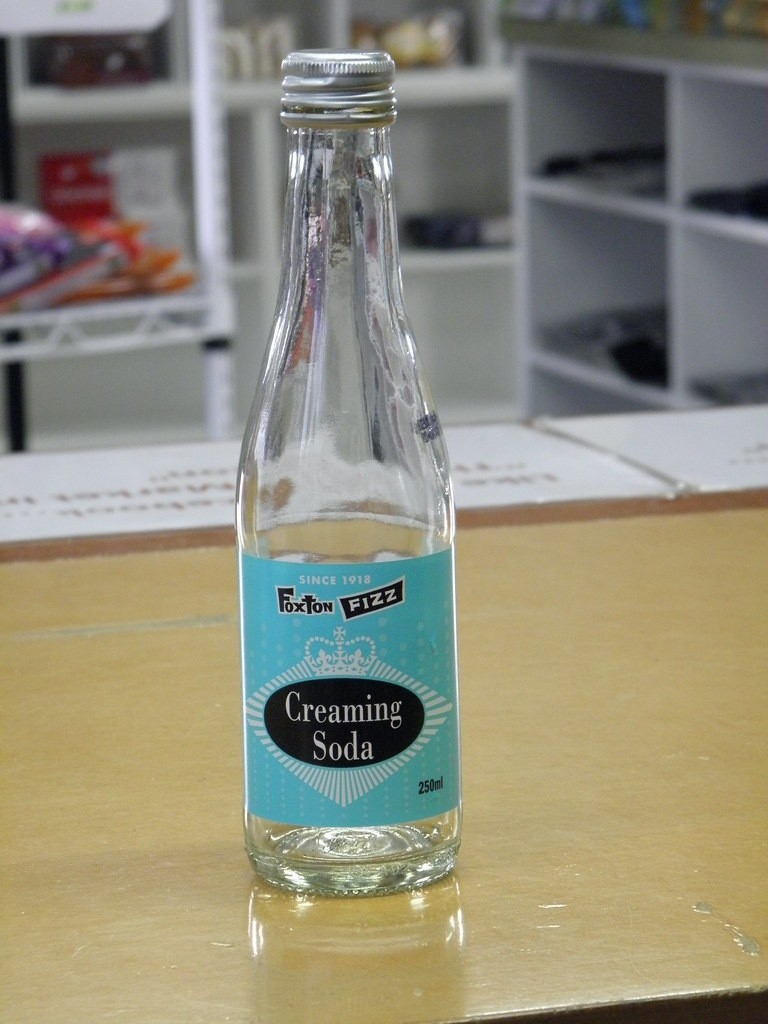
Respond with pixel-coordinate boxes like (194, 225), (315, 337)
(0, 404), (767, 1024)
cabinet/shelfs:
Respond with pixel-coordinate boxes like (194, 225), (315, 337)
(2, 0), (766, 452)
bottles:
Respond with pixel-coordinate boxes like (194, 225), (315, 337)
(234, 48), (462, 899)
